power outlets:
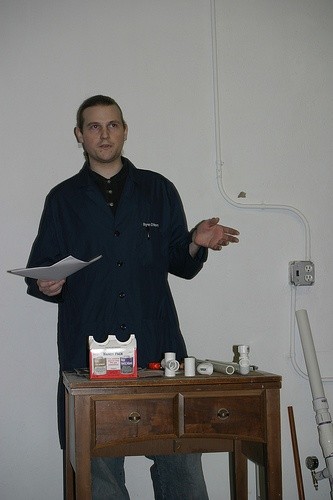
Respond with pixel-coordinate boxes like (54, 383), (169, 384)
(289, 261), (316, 286)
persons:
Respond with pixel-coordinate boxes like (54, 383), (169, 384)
(24, 94), (240, 499)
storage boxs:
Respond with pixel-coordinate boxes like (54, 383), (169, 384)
(89, 334), (138, 380)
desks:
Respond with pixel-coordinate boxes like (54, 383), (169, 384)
(61, 369), (283, 500)
